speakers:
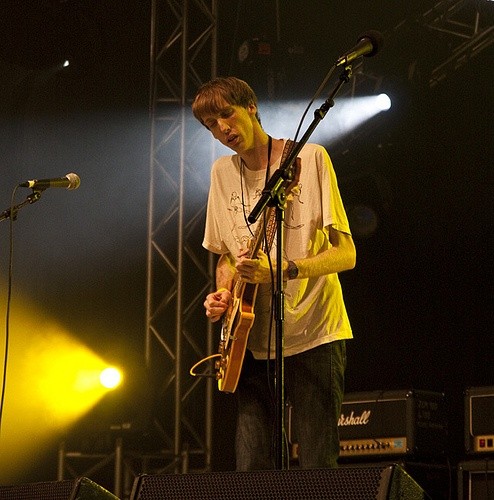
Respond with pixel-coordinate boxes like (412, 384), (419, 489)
(0, 478), (120, 500)
(456, 459), (494, 500)
(129, 462), (430, 500)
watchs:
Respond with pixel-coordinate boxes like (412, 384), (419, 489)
(286, 260), (298, 280)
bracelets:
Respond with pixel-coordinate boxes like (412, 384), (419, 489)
(217, 288), (229, 292)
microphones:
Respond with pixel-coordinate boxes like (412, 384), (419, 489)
(333, 30), (385, 67)
(20, 172), (81, 190)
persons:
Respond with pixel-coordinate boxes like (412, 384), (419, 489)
(192, 77), (357, 474)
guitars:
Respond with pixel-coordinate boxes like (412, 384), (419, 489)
(216, 137), (301, 393)
